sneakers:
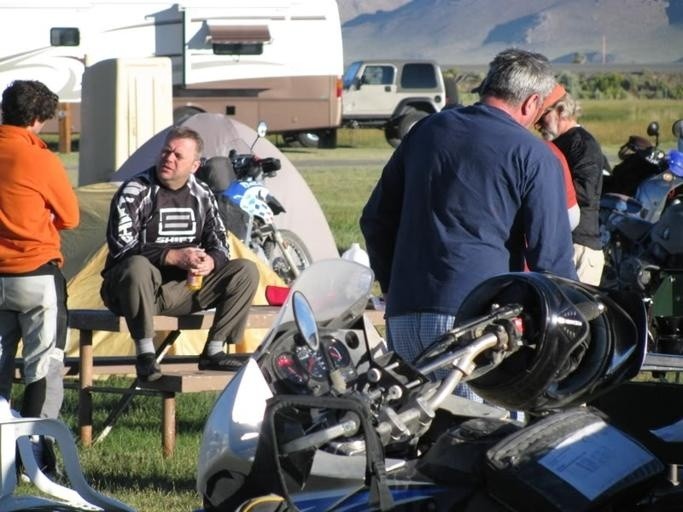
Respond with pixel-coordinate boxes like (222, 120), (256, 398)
(197, 349), (245, 372)
(21, 469), (64, 486)
(134, 352), (170, 388)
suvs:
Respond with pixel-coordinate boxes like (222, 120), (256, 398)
(278, 53), (460, 147)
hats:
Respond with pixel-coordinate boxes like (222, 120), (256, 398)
(532, 84), (568, 124)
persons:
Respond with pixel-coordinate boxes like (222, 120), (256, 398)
(100, 127), (259, 383)
(359, 50), (578, 426)
(542, 138), (581, 230)
(1, 80), (78, 484)
(529, 81), (604, 292)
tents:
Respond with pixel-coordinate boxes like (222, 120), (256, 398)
(13, 180), (290, 379)
(107, 112), (340, 264)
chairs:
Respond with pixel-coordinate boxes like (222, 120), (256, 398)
(0, 416), (135, 512)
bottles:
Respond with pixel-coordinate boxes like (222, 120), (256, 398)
(188, 265), (203, 291)
(341, 241), (370, 268)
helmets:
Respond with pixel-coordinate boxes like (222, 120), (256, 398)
(451, 268), (653, 419)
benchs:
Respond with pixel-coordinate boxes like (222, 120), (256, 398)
(135, 366), (241, 461)
(1, 302), (390, 447)
